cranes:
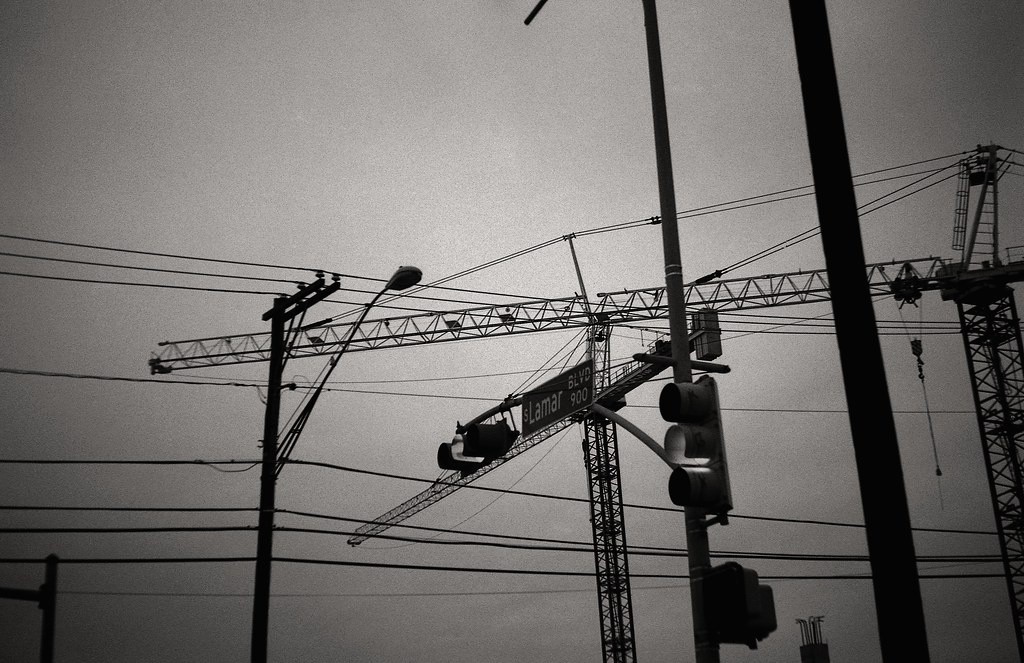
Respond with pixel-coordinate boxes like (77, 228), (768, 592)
(348, 308), (721, 662)
(147, 142), (1024, 663)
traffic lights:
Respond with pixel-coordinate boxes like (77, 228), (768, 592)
(706, 561), (778, 651)
(659, 375), (733, 517)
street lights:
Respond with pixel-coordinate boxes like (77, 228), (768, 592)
(249, 261), (427, 662)
